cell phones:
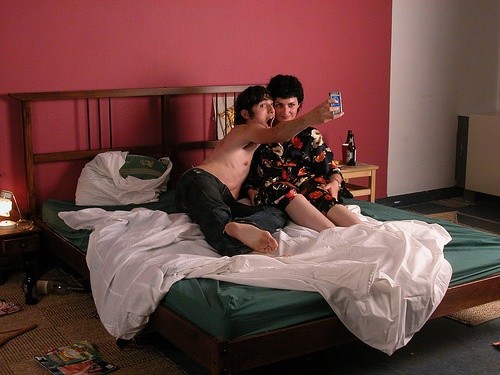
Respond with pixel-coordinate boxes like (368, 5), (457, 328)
(329, 91), (342, 115)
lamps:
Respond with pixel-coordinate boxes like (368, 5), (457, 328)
(0, 190), (34, 231)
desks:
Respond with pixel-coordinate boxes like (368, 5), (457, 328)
(336, 161), (379, 202)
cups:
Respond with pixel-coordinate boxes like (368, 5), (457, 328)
(342, 143), (349, 163)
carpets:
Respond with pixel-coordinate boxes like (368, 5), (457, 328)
(0, 264), (188, 375)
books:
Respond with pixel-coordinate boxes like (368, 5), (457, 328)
(33, 340), (116, 375)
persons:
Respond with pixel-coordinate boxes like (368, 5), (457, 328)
(242, 75), (361, 232)
(182, 85), (344, 255)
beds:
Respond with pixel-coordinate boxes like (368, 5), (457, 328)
(8, 83), (500, 375)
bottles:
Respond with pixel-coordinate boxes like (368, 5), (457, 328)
(346, 134), (357, 166)
(36, 280), (84, 294)
(345, 130), (352, 144)
(24, 269), (38, 305)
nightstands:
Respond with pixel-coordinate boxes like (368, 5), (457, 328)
(0, 222), (43, 274)
